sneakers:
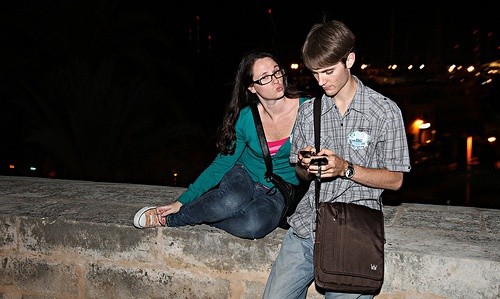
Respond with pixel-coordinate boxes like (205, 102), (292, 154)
(134, 207), (166, 229)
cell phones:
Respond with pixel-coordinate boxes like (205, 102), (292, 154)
(300, 151), (327, 166)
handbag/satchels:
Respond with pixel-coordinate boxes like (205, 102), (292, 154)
(249, 102), (305, 229)
(313, 95), (386, 294)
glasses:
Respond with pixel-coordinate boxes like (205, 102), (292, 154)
(252, 68), (286, 85)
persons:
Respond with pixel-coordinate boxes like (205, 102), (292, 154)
(133, 53), (313, 239)
(262, 20), (412, 299)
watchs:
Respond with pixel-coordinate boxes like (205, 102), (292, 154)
(340, 160), (355, 179)
